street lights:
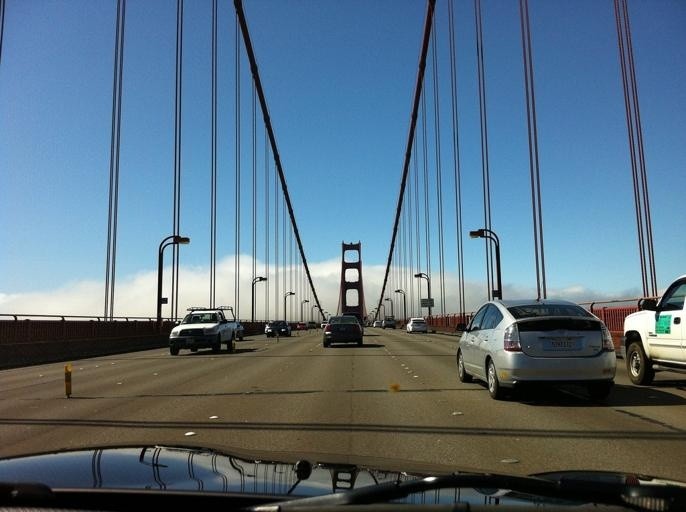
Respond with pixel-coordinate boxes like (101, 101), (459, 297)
(311, 305), (331, 328)
(470, 229), (502, 300)
(156, 235), (190, 345)
(395, 289), (406, 324)
(367, 303), (385, 321)
(284, 291), (295, 323)
(301, 300), (309, 322)
(415, 272), (431, 325)
(385, 298), (393, 316)
(251, 277), (267, 335)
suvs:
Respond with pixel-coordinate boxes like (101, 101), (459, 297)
(623, 274), (686, 385)
(168, 306), (237, 355)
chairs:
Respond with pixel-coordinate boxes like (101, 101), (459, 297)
(191, 314), (202, 325)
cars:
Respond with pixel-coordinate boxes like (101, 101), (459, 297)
(455, 299), (617, 400)
(320, 315), (364, 347)
(406, 317), (427, 334)
(308, 321), (317, 329)
(364, 316), (396, 329)
(265, 321), (291, 337)
(237, 322), (245, 341)
(296, 320), (308, 331)
